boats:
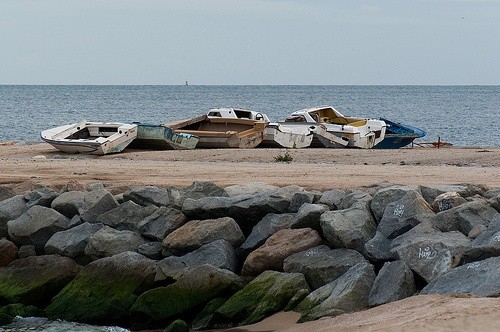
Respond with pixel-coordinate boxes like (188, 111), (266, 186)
(39, 105), (425, 157)
